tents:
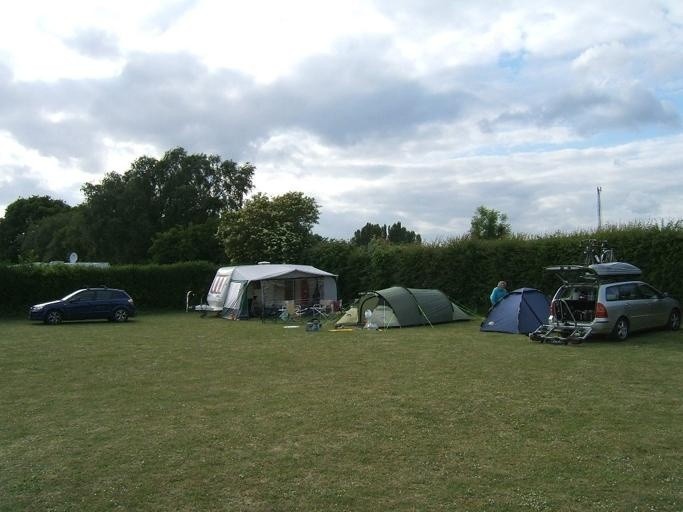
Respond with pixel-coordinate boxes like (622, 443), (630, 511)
(207, 264), (340, 321)
(480, 286), (552, 335)
(356, 282), (475, 328)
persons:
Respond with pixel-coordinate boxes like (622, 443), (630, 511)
(489, 280), (509, 305)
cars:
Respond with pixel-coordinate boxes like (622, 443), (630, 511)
(529, 239), (683, 344)
(28, 285), (135, 325)
(611, 340), (613, 342)
